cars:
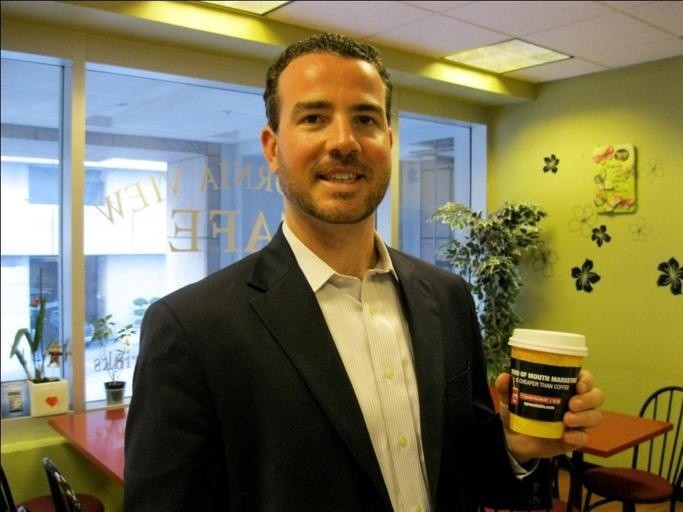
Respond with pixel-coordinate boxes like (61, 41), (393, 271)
(30, 287), (94, 361)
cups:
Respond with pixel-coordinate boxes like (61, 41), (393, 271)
(508, 328), (589, 439)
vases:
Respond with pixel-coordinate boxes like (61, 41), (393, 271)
(27, 377), (67, 418)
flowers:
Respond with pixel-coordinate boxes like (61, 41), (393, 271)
(9, 269), (63, 380)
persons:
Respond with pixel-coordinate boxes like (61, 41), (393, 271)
(119, 29), (607, 511)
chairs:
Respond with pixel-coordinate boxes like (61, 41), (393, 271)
(0, 457), (106, 512)
(574, 384), (683, 512)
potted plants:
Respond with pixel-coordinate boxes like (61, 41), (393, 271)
(92, 315), (137, 405)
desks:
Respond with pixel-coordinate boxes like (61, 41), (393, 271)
(50, 407), (138, 483)
(560, 407), (673, 512)
